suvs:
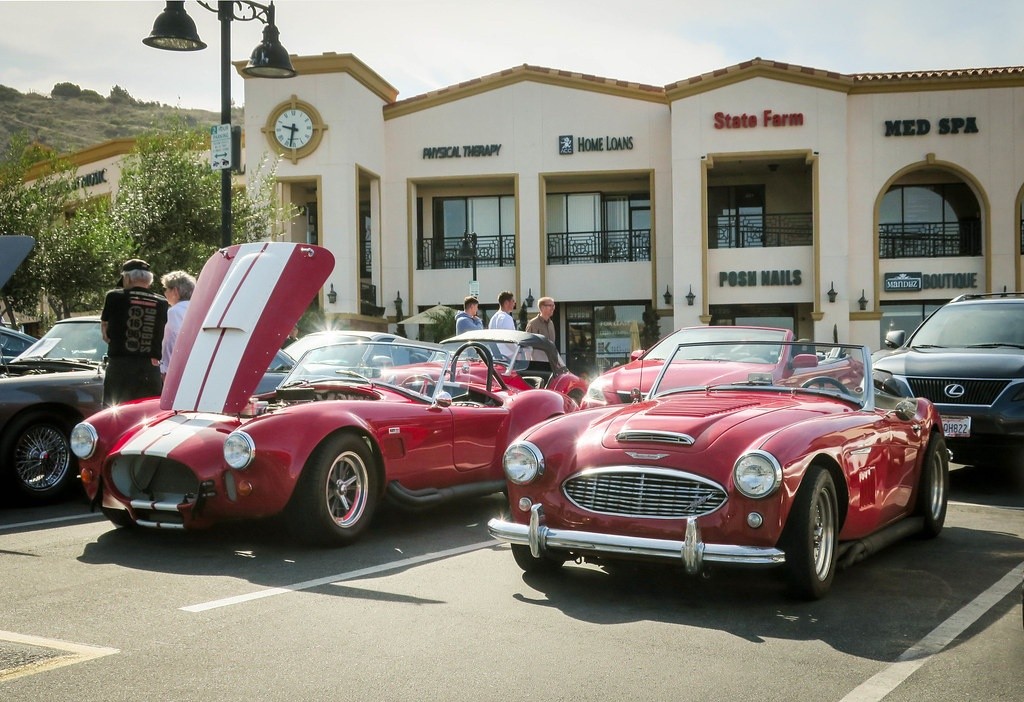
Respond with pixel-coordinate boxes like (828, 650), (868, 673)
(872, 292), (1023, 475)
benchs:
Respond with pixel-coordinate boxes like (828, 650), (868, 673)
(408, 384), (501, 408)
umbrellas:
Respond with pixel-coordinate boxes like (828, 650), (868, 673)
(397, 305), (459, 324)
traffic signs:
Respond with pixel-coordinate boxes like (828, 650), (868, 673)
(209, 124), (233, 170)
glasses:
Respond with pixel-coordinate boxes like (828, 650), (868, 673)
(162, 286), (168, 292)
(544, 304), (555, 308)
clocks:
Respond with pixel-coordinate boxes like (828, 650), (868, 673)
(261, 94), (329, 164)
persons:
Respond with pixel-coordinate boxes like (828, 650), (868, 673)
(455, 297), (483, 354)
(489, 291), (521, 360)
(102, 260), (168, 409)
(160, 271), (196, 381)
(281, 325), (298, 349)
(525, 297), (555, 363)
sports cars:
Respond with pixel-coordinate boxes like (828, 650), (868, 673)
(580, 325), (865, 410)
(487, 340), (954, 606)
(70, 241), (584, 555)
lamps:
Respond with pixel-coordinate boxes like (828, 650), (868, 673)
(686, 285), (696, 305)
(828, 281), (838, 302)
(328, 284), (337, 303)
(394, 291), (401, 309)
(526, 288), (534, 307)
(663, 285), (672, 304)
(858, 289), (868, 310)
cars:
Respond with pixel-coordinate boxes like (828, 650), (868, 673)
(0, 233), (40, 362)
(282, 328), (588, 406)
(14, 313), (312, 395)
(1, 315), (108, 509)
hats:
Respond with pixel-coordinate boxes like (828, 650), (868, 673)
(116, 259), (151, 288)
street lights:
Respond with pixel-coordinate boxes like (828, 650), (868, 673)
(141, 1), (298, 244)
